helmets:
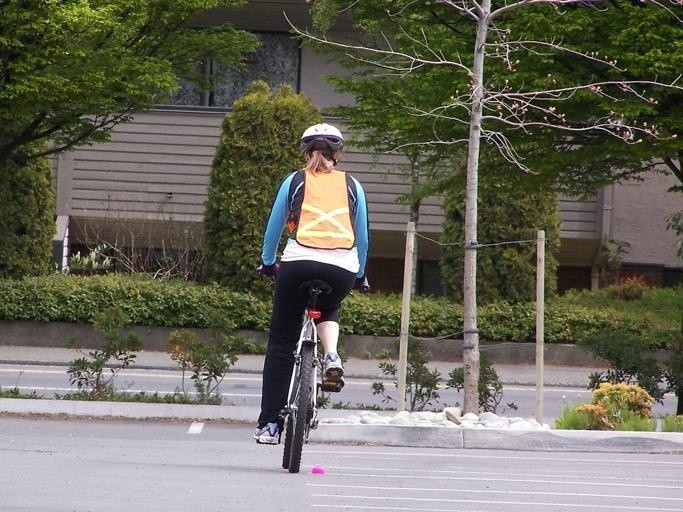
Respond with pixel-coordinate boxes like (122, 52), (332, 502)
(301, 123), (344, 151)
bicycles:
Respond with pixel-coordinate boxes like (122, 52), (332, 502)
(256, 265), (370, 473)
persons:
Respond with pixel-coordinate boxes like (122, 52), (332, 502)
(254, 122), (370, 445)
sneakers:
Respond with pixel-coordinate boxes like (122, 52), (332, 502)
(253, 422), (281, 444)
(321, 351), (345, 389)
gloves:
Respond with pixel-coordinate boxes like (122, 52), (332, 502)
(256, 261), (280, 279)
(355, 277), (370, 292)
